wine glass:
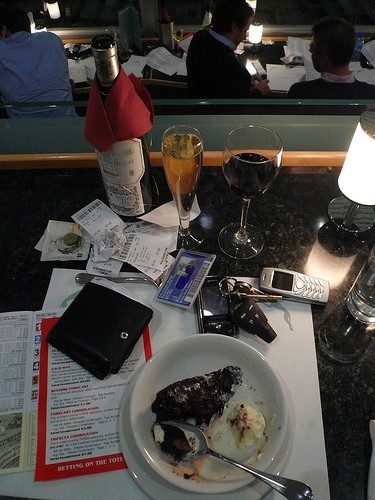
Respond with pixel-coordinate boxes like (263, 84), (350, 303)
(172, 24), (184, 56)
(161, 125), (206, 251)
(218, 125), (284, 259)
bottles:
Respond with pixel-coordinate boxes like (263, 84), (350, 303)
(355, 31), (365, 60)
(92, 33), (160, 221)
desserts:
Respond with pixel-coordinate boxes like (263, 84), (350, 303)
(151, 366), (266, 448)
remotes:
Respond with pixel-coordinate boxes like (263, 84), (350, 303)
(251, 61), (265, 76)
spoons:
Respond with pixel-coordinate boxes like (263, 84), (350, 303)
(155, 421), (313, 500)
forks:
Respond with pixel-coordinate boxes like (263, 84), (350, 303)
(75, 272), (222, 290)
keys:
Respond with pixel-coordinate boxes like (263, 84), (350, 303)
(218, 277), (283, 344)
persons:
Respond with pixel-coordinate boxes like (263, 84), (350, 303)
(184, 1), (270, 101)
(286, 17), (375, 101)
(1, 8), (78, 116)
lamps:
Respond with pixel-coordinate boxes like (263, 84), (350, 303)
(34, 24), (47, 33)
(249, 9), (264, 44)
(43, 0), (61, 19)
(327, 106), (375, 234)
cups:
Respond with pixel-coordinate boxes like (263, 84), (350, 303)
(117, 33), (130, 60)
(347, 243), (375, 324)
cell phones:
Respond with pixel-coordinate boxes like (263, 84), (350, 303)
(194, 275), (239, 338)
(259, 267), (330, 306)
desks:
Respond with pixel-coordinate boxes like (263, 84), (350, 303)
(62, 41), (375, 95)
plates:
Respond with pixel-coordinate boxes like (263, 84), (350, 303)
(119, 361), (293, 500)
(130, 333), (288, 493)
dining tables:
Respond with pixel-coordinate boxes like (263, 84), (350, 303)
(0, 165), (374, 500)
